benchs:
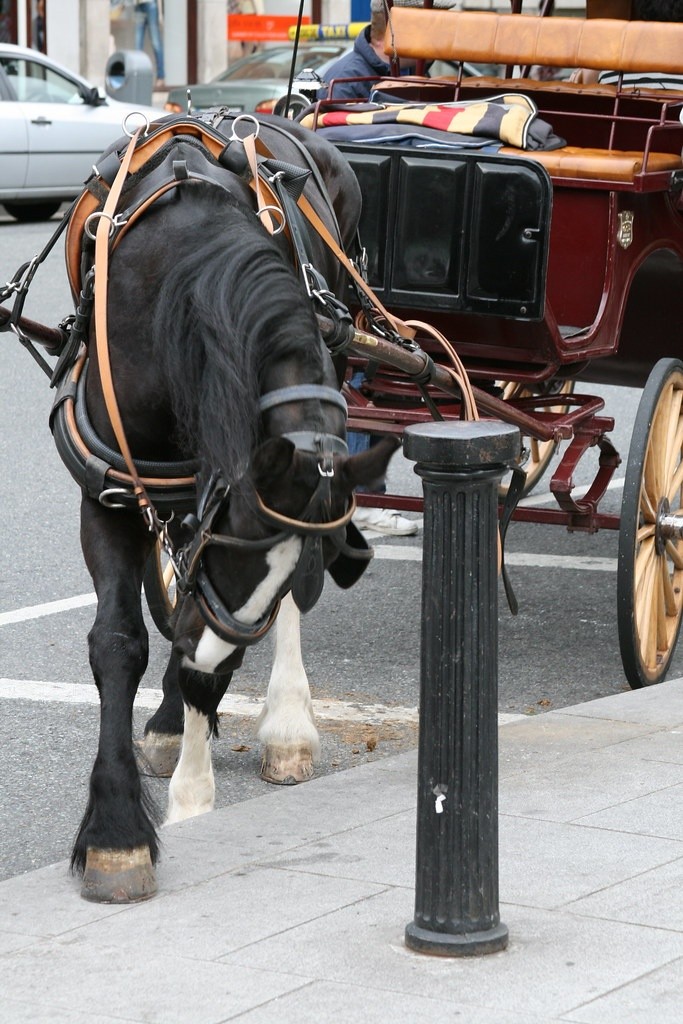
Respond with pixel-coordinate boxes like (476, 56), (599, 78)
(294, 7), (683, 193)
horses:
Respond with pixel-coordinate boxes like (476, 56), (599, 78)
(52, 108), (404, 908)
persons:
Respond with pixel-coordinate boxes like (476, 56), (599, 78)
(316, 0), (456, 537)
(570, 65), (682, 90)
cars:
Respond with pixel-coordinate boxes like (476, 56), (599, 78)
(166, 23), (370, 119)
(1, 44), (177, 223)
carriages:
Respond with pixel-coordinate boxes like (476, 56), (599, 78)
(0, 1), (683, 903)
